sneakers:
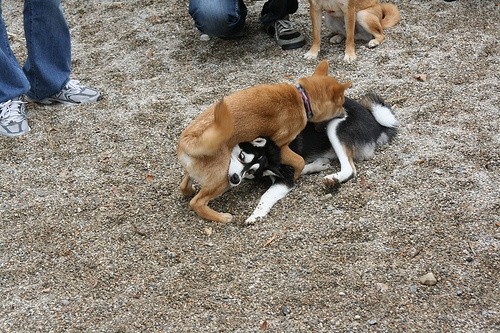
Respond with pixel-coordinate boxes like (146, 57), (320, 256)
(261, 15), (306, 50)
(24, 79), (103, 106)
(0, 94), (31, 138)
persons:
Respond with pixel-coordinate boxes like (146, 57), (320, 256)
(0, 0), (102, 137)
(187, 0), (307, 50)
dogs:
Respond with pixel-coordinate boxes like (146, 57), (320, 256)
(178, 60), (400, 226)
(304, 0), (401, 63)
